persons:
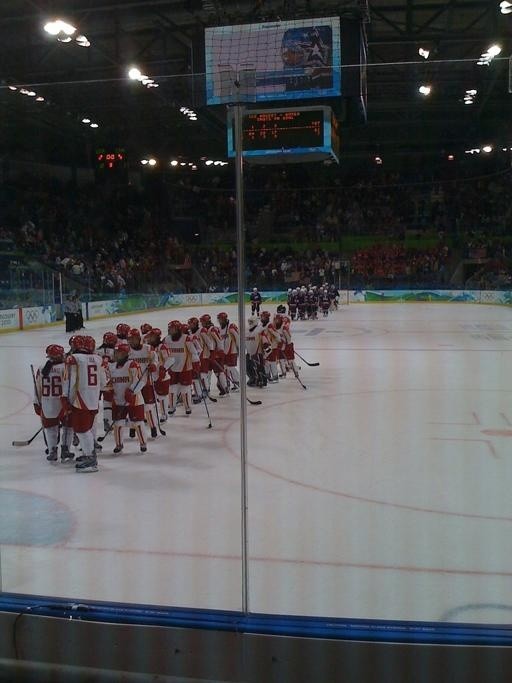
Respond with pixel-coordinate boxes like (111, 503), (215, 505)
(248, 286), (262, 318)
(287, 281), (340, 321)
(242, 168), (511, 297)
(59, 292), (87, 334)
(33, 303), (298, 469)
(0, 168), (238, 297)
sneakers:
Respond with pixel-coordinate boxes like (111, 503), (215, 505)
(160, 414), (167, 424)
(150, 426), (156, 438)
(130, 427), (135, 436)
(168, 407), (175, 414)
(104, 418), (113, 430)
(46, 434), (102, 467)
(113, 443), (123, 452)
(140, 442), (146, 451)
(186, 407), (191, 413)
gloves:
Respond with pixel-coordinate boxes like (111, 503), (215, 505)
(148, 364), (156, 372)
(124, 388), (133, 402)
(33, 403), (41, 414)
(60, 396), (69, 410)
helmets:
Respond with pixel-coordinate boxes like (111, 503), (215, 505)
(217, 312), (227, 320)
(103, 323), (161, 352)
(277, 304), (286, 312)
(168, 313), (212, 333)
(247, 310), (283, 329)
(46, 335), (95, 358)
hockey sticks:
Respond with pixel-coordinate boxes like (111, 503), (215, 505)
(201, 379), (217, 401)
(281, 350), (311, 389)
(150, 372), (166, 436)
(12, 426), (45, 447)
(98, 361), (151, 442)
(214, 360), (262, 405)
(197, 375), (212, 428)
(293, 350), (319, 366)
